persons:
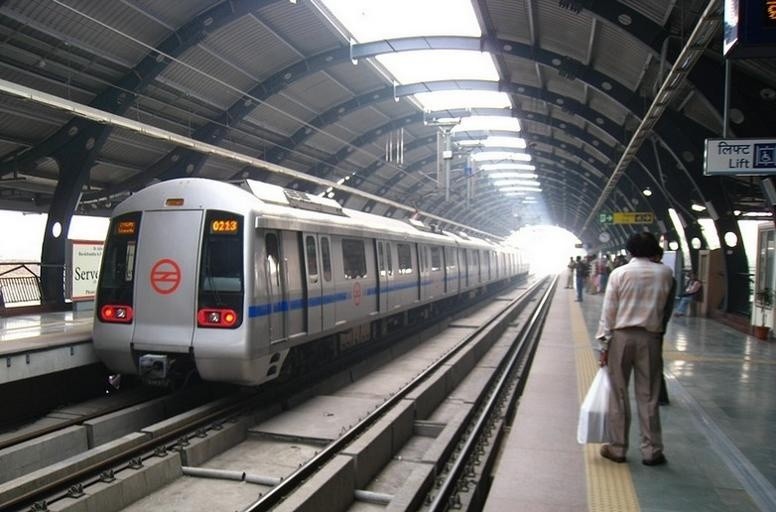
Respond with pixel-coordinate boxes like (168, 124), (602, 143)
(675, 271), (704, 315)
(595, 232), (673, 466)
(649, 246), (677, 406)
(565, 253), (627, 301)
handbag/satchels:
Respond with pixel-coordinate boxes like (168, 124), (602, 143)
(577, 365), (611, 444)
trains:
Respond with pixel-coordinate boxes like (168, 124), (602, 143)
(92, 177), (529, 399)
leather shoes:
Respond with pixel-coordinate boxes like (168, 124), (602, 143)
(600, 445), (626, 463)
(642, 455), (667, 466)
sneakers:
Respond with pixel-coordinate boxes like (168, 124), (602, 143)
(674, 312), (684, 318)
